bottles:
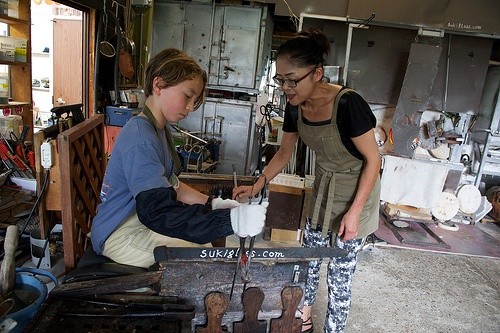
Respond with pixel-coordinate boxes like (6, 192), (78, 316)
(38, 118), (41, 126)
(54, 117), (58, 125)
(48, 115), (53, 126)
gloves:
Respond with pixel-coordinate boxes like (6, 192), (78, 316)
(212, 197), (243, 210)
(230, 201), (269, 238)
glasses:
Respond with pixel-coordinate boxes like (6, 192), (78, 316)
(272, 66), (317, 87)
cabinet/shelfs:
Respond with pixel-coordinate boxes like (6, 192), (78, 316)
(32, 53), (50, 91)
(0, 0), (36, 214)
(167, 97), (262, 186)
(150, 2), (274, 93)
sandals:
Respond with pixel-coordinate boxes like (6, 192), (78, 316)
(302, 316), (314, 332)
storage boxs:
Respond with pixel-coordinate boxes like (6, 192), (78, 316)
(0, 35), (28, 63)
(0, 0), (19, 18)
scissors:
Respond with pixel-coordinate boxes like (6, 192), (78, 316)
(259, 102), (273, 134)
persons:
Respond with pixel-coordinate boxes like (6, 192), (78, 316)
(90, 48), (269, 269)
(232, 27), (381, 333)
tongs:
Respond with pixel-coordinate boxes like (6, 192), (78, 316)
(55, 297), (195, 320)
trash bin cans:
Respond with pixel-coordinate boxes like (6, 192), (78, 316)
(1, 269), (50, 331)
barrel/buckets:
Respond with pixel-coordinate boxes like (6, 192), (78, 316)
(0, 267), (58, 333)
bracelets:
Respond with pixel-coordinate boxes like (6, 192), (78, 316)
(205, 195), (215, 210)
